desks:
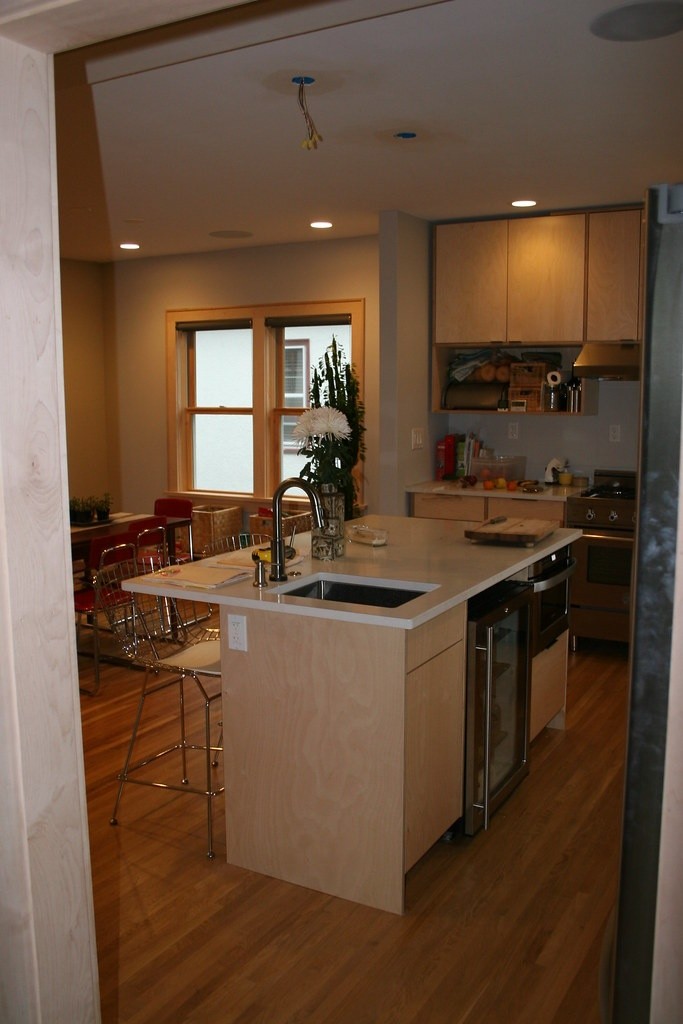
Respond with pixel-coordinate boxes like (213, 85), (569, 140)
(70, 511), (192, 645)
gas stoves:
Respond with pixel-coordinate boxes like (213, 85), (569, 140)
(567, 470), (637, 529)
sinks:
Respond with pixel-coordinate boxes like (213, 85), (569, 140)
(266, 571), (442, 608)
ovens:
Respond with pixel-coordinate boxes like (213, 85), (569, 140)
(570, 529), (634, 651)
(528, 545), (577, 656)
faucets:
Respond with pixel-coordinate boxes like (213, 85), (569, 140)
(269, 478), (325, 581)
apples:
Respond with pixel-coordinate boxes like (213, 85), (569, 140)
(467, 364), (510, 382)
(480, 469), (518, 491)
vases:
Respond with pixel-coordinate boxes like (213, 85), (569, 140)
(309, 482), (348, 561)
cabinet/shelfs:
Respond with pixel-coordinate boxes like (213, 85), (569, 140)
(406, 480), (588, 529)
(428, 202), (647, 416)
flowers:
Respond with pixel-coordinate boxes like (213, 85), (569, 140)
(291, 405), (353, 483)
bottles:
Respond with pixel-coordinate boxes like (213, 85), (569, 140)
(455, 434), (465, 477)
(561, 383), (568, 411)
(571, 384), (579, 413)
(544, 384), (559, 411)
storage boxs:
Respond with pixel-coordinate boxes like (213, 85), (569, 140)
(181, 503), (242, 555)
(249, 508), (311, 547)
(471, 455), (526, 483)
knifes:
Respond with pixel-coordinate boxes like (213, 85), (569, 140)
(472, 517), (507, 532)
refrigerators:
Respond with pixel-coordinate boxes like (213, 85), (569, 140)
(467, 582), (533, 836)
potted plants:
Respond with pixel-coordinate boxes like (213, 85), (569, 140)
(70, 493), (113, 523)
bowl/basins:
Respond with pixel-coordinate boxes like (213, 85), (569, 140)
(471, 456), (527, 489)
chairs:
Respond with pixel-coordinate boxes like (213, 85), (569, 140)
(73, 497), (275, 858)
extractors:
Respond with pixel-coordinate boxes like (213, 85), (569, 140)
(572, 344), (640, 381)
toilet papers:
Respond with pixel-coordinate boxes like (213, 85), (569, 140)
(547, 370), (571, 385)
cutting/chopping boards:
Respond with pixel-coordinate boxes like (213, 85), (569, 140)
(464, 516), (560, 543)
